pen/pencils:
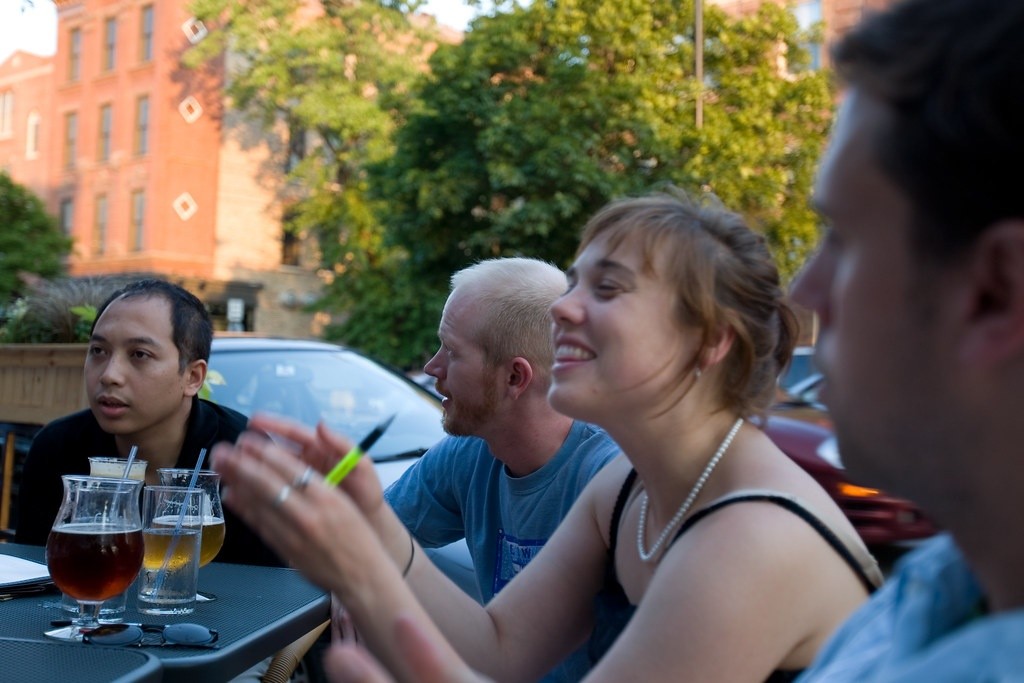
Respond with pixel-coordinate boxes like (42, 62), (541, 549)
(49, 620), (219, 633)
(323, 412), (400, 489)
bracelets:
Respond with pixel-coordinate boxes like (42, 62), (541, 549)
(401, 528), (415, 577)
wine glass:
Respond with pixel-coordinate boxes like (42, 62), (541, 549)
(46, 475), (144, 645)
(155, 469), (226, 601)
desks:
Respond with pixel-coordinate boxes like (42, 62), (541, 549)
(0, 542), (335, 683)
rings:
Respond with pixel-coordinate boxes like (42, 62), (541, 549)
(273, 485), (291, 510)
(295, 465), (314, 491)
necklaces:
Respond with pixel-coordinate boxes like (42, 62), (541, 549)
(634, 417), (745, 562)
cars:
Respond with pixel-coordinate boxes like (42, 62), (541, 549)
(746, 345), (944, 545)
(195, 334), (489, 611)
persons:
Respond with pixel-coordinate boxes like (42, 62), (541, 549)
(12, 279), (291, 683)
(210, 0), (1024, 683)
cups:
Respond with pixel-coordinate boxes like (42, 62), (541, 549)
(63, 486), (137, 622)
(140, 486), (204, 615)
(89, 456), (147, 484)
